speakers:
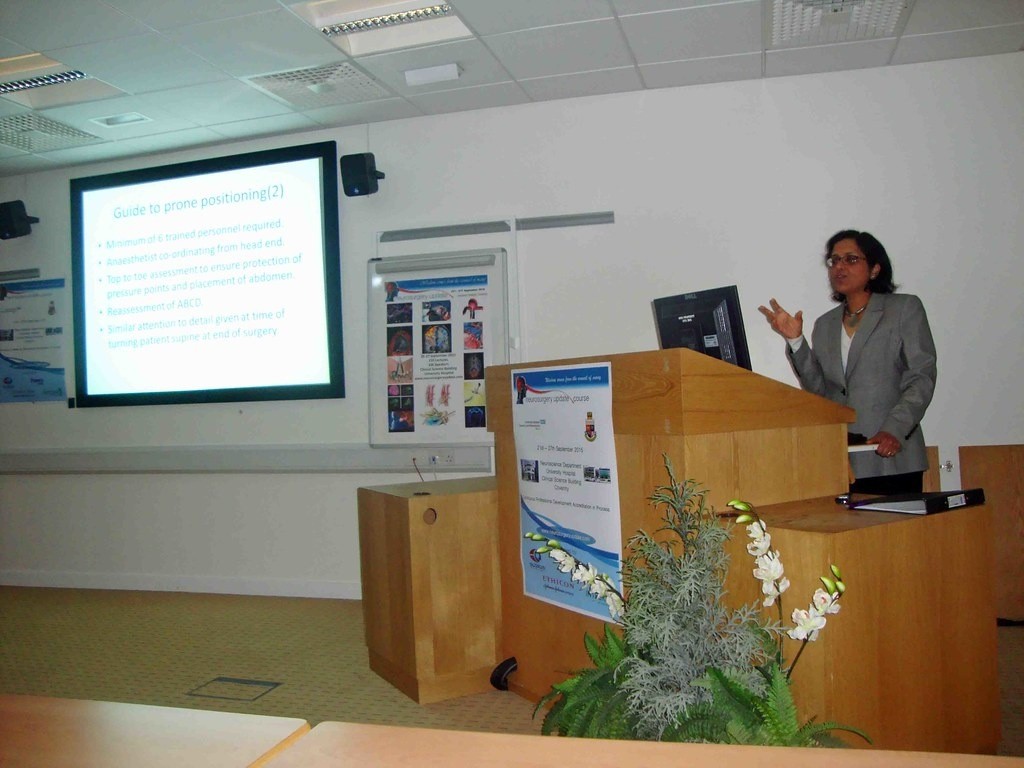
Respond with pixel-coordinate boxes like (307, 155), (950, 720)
(0, 200), (32, 241)
(340, 152), (378, 197)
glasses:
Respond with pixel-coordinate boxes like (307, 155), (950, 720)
(826, 255), (866, 267)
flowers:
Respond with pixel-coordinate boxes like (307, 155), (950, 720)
(526, 447), (875, 747)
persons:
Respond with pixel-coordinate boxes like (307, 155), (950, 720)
(758, 230), (938, 493)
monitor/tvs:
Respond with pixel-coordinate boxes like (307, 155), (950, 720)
(653, 285), (752, 371)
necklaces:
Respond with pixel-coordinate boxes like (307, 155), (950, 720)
(845, 305), (866, 327)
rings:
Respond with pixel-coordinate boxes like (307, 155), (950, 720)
(888, 452), (891, 454)
